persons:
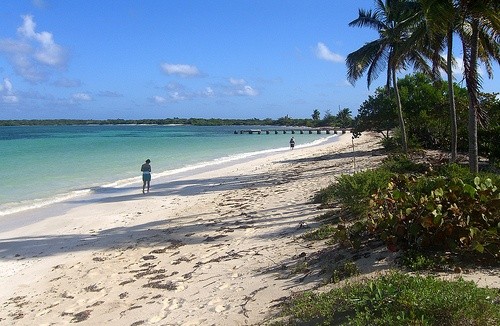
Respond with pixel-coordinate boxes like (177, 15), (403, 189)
(141, 159), (151, 194)
(290, 137), (296, 149)
